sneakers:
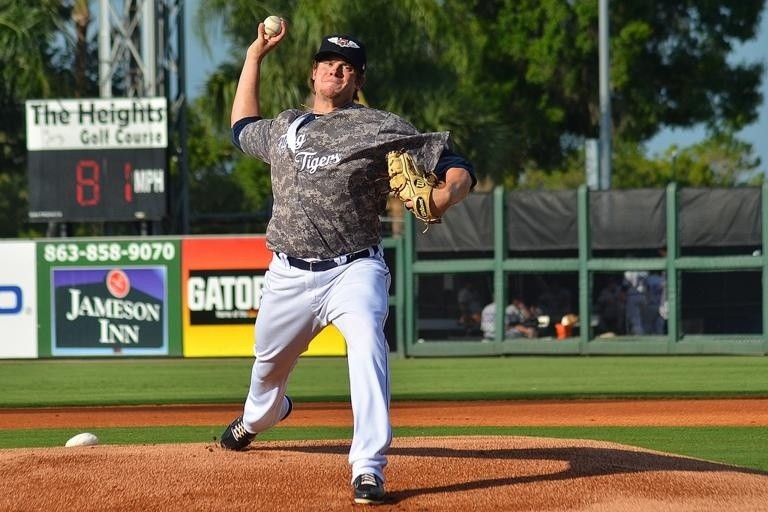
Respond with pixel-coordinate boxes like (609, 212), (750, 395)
(353, 473), (385, 504)
(221, 395), (292, 450)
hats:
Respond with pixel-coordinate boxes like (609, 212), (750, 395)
(313, 35), (366, 74)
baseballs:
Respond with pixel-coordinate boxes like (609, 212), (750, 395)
(263, 16), (282, 36)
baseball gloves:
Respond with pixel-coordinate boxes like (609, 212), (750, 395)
(387, 147), (442, 224)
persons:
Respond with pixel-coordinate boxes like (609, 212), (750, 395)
(480, 292), (519, 341)
(596, 248), (674, 339)
(508, 294), (536, 338)
(537, 273), (574, 320)
(221, 20), (478, 504)
(458, 276), (481, 340)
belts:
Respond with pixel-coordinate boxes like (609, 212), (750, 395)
(276, 246), (379, 271)
